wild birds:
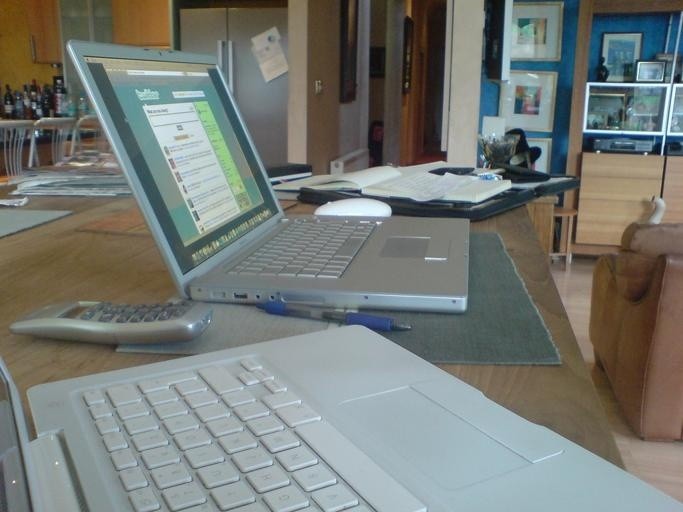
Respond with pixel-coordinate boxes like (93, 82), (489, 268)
(65, 39), (471, 312)
(0, 324), (683, 512)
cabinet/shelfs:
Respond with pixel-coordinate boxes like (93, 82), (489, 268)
(5, 78), (87, 122)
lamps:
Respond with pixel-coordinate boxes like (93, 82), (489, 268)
(1, 110), (114, 176)
(590, 214), (683, 443)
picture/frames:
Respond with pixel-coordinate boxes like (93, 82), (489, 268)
(3, 164), (626, 476)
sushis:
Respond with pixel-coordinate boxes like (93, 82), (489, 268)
(8, 298), (214, 341)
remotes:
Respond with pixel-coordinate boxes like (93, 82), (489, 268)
(314, 198), (392, 218)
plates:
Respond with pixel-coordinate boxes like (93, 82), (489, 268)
(562, 0), (683, 259)
(110, 0), (181, 47)
(25, 0), (62, 65)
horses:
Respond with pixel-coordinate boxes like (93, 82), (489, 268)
(9, 300), (215, 345)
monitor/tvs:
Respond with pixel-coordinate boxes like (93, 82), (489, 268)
(272, 160), (514, 204)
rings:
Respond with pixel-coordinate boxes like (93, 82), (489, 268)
(479, 134), (515, 167)
(257, 301), (412, 332)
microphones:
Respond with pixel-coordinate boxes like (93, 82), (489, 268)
(551, 205), (579, 272)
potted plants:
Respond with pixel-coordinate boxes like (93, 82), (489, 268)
(599, 30), (668, 83)
(507, 0), (565, 175)
